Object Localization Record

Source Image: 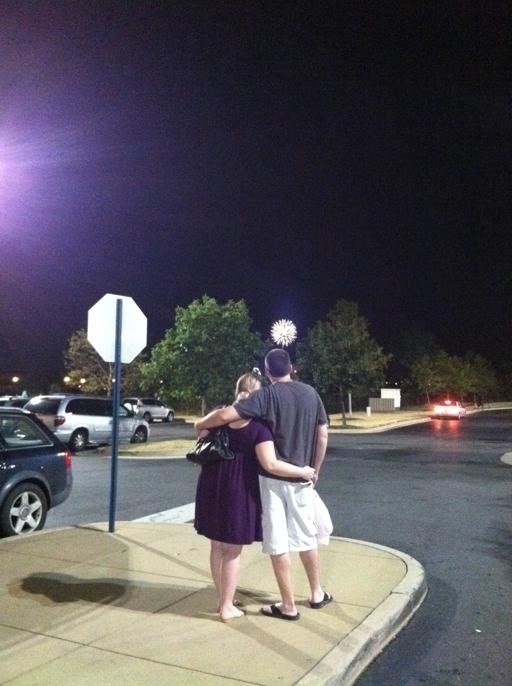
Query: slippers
[259,602,298,621]
[309,591,333,608]
[218,598,247,621]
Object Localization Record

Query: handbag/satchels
[186,427,236,465]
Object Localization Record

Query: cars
[431,400,467,420]
[0,392,179,450]
[0,404,76,537]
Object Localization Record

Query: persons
[193,349,333,621]
[193,374,316,624]
